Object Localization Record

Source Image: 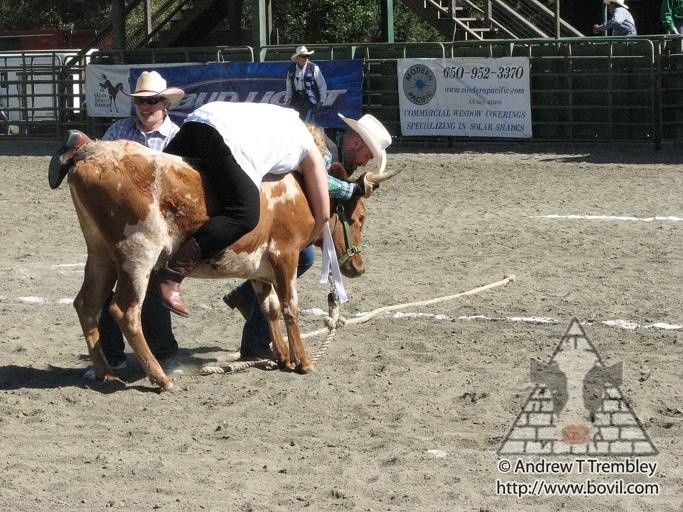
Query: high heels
[222,280,257,321]
[47,129,93,190]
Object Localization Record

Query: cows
[66,138,408,396]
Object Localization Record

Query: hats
[603,0,629,10]
[338,112,393,174]
[290,45,315,63]
[121,70,185,104]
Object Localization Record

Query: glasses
[134,97,163,105]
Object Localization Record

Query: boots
[150,236,204,318]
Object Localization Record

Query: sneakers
[83,359,128,380]
[159,358,185,377]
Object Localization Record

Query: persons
[83,70,185,382]
[659,0,683,72]
[149,99,332,318]
[222,111,393,361]
[284,45,328,123]
[592,0,638,44]
